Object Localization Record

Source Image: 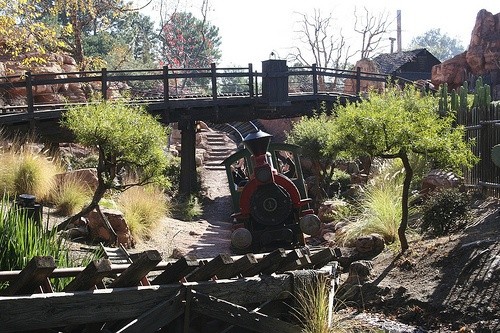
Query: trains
[222,127,321,254]
